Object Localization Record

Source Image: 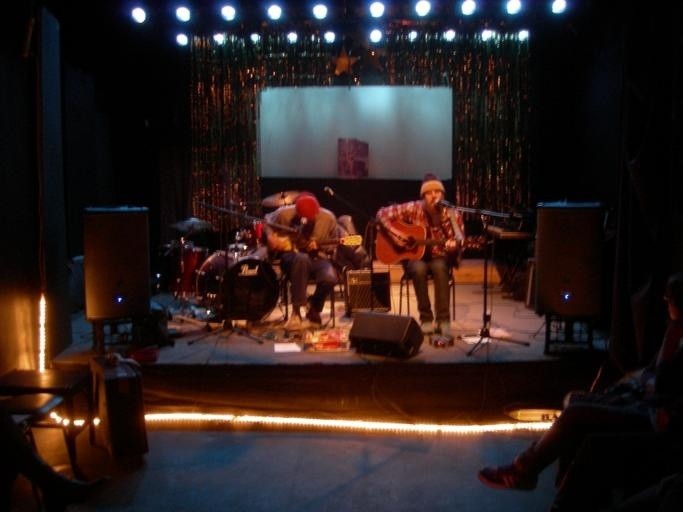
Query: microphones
[434,198,457,210]
[294,216,310,233]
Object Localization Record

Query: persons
[0,407,111,512]
[264,196,338,331]
[377,173,465,334]
[478,281,683,492]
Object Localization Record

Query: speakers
[130,310,168,348]
[349,312,424,358]
[346,270,391,318]
[533,202,607,321]
[83,207,152,320]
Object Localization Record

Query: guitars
[267,230,363,260]
[376,222,481,265]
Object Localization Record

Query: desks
[0,368,95,482]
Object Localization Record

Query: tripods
[188,203,296,345]
[457,235,531,357]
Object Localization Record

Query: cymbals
[262,191,302,207]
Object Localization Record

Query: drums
[195,251,278,320]
[174,248,211,301]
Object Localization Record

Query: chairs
[397,267,455,324]
[281,274,336,329]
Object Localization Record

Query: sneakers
[477,454,538,491]
[284,308,323,329]
[421,318,449,339]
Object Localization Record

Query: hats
[418,172,446,196]
[296,191,320,218]
